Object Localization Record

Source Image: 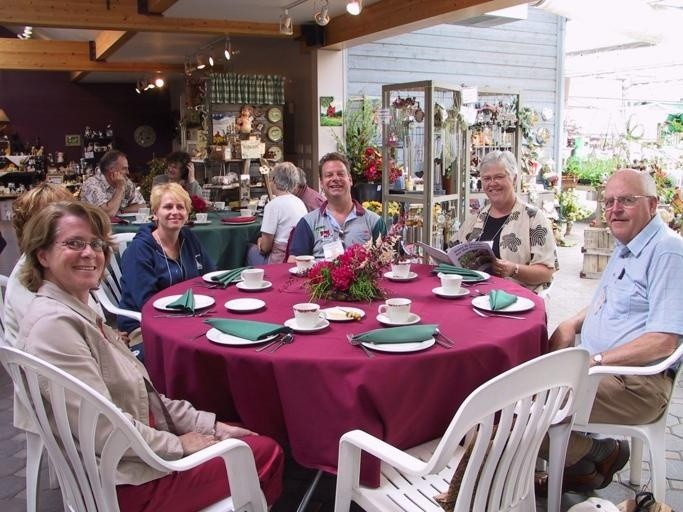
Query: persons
[517,169,683,497]
[287,153,387,264]
[151,155,205,216]
[248,160,327,267]
[116,181,214,353]
[83,155,144,222]
[4,186,132,372]
[448,151,554,295]
[12,200,284,512]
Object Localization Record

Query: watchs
[593,352,605,366]
[510,263,520,278]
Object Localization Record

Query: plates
[131,220,151,225]
[288,267,306,276]
[236,280,273,291]
[432,286,471,298]
[134,125,156,148]
[267,108,283,162]
[319,306,366,322]
[202,270,242,282]
[437,270,491,283]
[192,220,212,225]
[225,298,266,312]
[152,294,216,312]
[359,335,436,352]
[383,271,419,281]
[375,313,421,326]
[284,317,330,333]
[206,327,279,346]
[471,295,535,312]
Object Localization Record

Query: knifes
[462,283,490,287]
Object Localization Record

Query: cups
[438,273,463,293]
[391,259,411,278]
[241,268,265,287]
[378,297,412,322]
[295,255,316,272]
[136,202,257,222]
[292,303,326,328]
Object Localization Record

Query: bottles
[26,124,113,188]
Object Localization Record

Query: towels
[488,289,517,312]
[433,263,483,282]
[352,323,439,344]
[221,215,255,223]
[203,316,297,342]
[112,216,129,226]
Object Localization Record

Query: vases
[358,183,382,203]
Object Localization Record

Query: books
[414,239,496,282]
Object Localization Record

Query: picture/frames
[65,134,80,147]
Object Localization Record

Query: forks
[346,332,376,359]
[473,308,527,321]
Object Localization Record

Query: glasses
[602,195,654,209]
[53,239,109,253]
[478,174,508,183]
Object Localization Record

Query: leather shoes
[535,465,604,496]
[597,439,631,490]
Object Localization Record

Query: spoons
[270,332,294,352]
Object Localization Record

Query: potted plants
[561,160,580,192]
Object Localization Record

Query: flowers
[329,96,402,183]
[187,195,214,219]
[307,202,446,301]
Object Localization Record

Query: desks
[142,257,547,512]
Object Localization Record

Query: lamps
[136,73,165,92]
[184,36,239,74]
[280,0,364,35]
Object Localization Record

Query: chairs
[571,339,683,503]
[335,346,589,512]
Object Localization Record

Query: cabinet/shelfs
[382,81,534,251]
